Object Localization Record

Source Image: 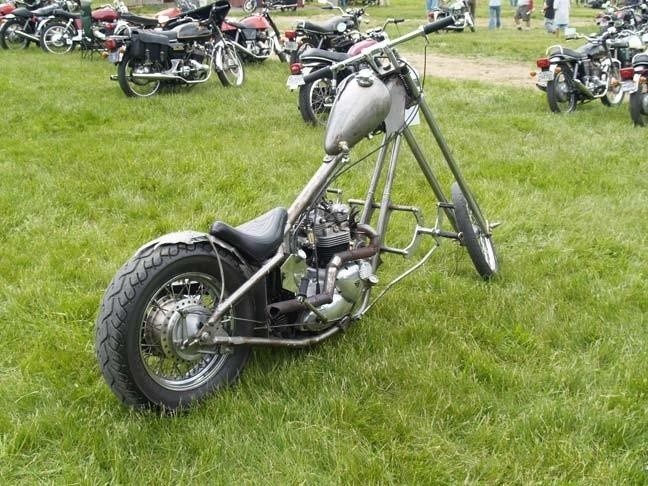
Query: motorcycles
[530,0,648,124]
[427,0,475,35]
[0,0,404,128]
[94,15,500,417]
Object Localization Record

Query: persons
[515,0,533,31]
[487,0,502,31]
[553,0,570,38]
[539,0,558,34]
